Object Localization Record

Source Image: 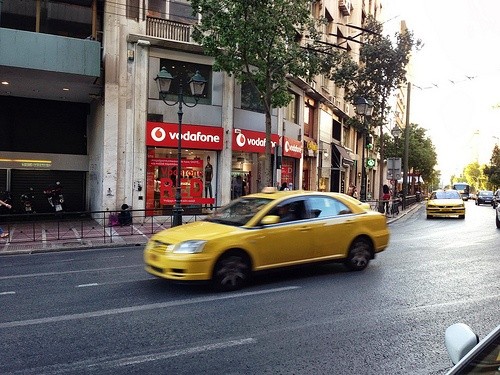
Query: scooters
[18,188,36,221]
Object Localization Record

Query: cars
[426,190,465,220]
[142,187,391,290]
[493,189,500,209]
[442,323,500,374]
[496,208,500,227]
[475,191,493,205]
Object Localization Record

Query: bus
[454,183,469,201]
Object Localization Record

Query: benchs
[377,198,403,214]
[405,195,416,210]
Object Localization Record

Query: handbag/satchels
[108,212,120,226]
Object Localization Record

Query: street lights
[153,65,207,224]
[353,96,376,199]
[391,123,400,201]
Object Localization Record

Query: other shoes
[0,232,9,238]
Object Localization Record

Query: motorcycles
[42,181,65,222]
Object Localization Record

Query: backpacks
[383,194,390,200]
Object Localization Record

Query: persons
[280,182,289,191]
[233,176,244,199]
[201,155,213,211]
[0,198,13,238]
